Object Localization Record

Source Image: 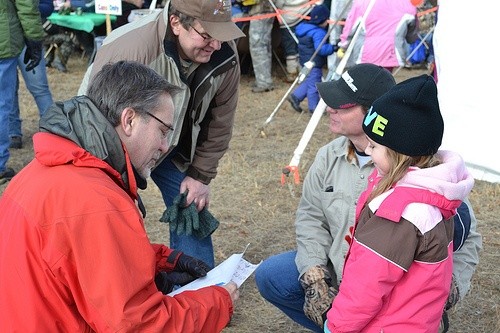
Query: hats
[308,5,329,25]
[171,0,246,42]
[362,74,444,156]
[315,63,396,110]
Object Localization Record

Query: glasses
[145,110,174,141]
[188,23,225,44]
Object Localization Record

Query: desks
[45,11,117,65]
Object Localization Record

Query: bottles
[76,7,82,16]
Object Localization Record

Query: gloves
[333,45,339,51]
[299,264,338,329]
[175,254,214,279]
[23,38,43,71]
[159,190,221,241]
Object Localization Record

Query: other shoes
[50,61,66,72]
[0,167,16,185]
[286,72,298,82]
[252,87,275,92]
[288,94,303,113]
[9,135,22,147]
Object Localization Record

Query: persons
[231,0,438,115]
[0,60,240,333]
[78,0,246,293]
[0,0,153,186]
[323,75,475,333]
[255,63,484,333]
